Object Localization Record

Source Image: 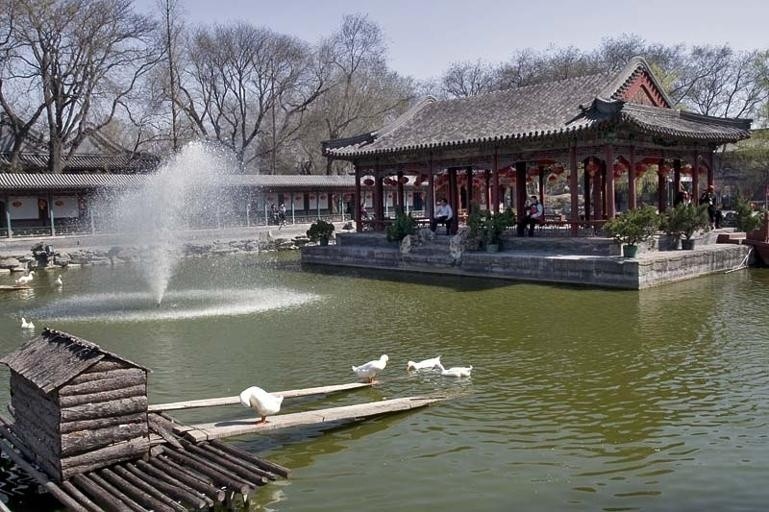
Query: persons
[516,196,544,237]
[270,204,280,225]
[278,203,288,225]
[673,183,722,229]
[430,198,453,236]
[361,202,376,231]
[749,201,761,214]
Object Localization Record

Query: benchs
[364,215,607,238]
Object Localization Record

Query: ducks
[406,355,442,371]
[21,317,35,329]
[15,271,36,287]
[239,385,283,425]
[55,274,63,286]
[352,354,389,383]
[432,363,473,377]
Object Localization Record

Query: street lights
[667,178,673,207]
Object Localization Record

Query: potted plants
[600,204,660,257]
[659,200,710,249]
